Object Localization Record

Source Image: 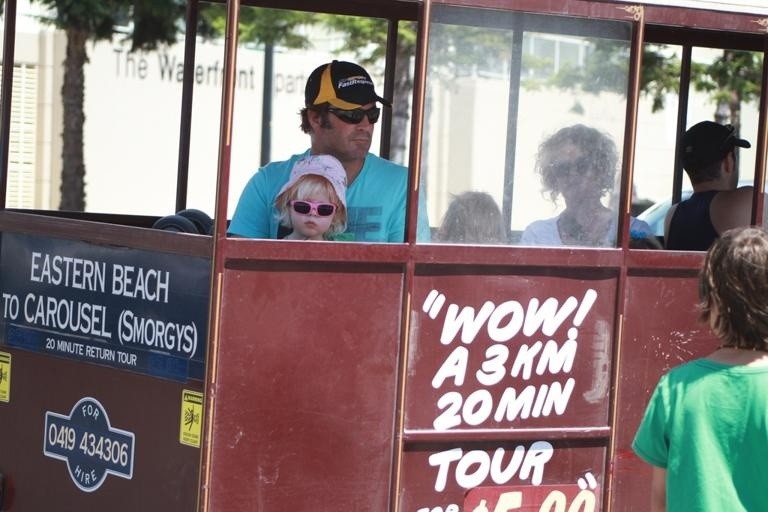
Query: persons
[518,125,663,248]
[663,121,767,251]
[226,59,431,245]
[435,190,510,244]
[627,226,768,511]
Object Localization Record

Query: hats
[677,121,751,169]
[304,60,392,112]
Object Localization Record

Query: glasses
[290,199,337,220]
[547,156,594,176]
[721,123,736,144]
[329,108,381,124]
